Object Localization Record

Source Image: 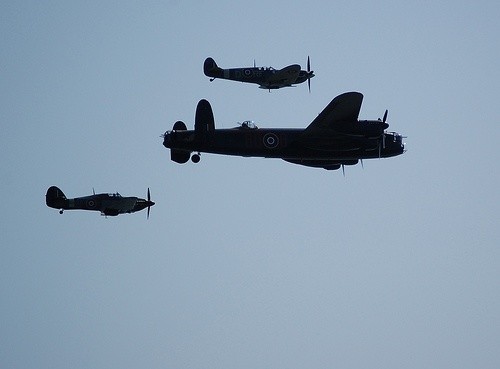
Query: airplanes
[203,55,315,92]
[160,91,407,175]
[46,186,155,219]
